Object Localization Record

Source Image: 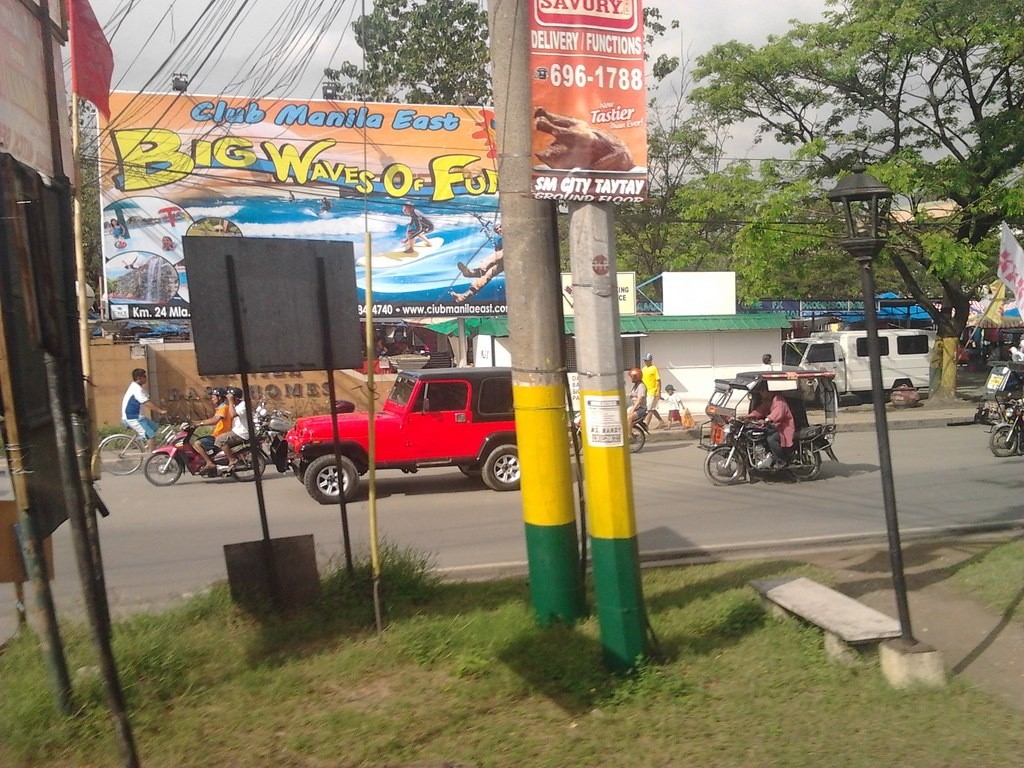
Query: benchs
[747,575,902,659]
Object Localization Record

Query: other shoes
[451,291,470,304]
[227,459,239,472]
[773,456,789,469]
[458,262,474,277]
[655,423,671,430]
[199,464,216,474]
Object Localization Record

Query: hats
[643,353,652,361]
[665,385,674,390]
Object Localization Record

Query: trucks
[782,330,935,395]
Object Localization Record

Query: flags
[997,220,1024,324]
[67,0,114,123]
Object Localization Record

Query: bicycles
[98,413,180,476]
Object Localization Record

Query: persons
[761,354,774,371]
[450,221,505,305]
[957,334,1024,373]
[109,219,127,239]
[737,382,795,469]
[363,329,421,358]
[641,353,665,431]
[189,385,231,473]
[660,384,689,430]
[120,368,169,465]
[625,367,647,440]
[402,202,435,253]
[223,387,249,473]
[161,237,177,252]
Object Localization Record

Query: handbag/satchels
[681,409,696,428]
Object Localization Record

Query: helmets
[209,387,242,400]
[628,368,642,382]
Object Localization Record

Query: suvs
[285,367,521,505]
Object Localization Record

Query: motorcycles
[143,422,269,487]
[704,415,838,486]
[567,412,650,456]
[254,400,354,446]
[990,393,1024,456]
[981,361,1024,424]
[700,374,839,451]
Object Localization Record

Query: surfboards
[356,237,444,268]
[309,207,320,217]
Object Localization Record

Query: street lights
[823,161,914,642]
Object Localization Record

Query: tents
[930,298,1024,337]
